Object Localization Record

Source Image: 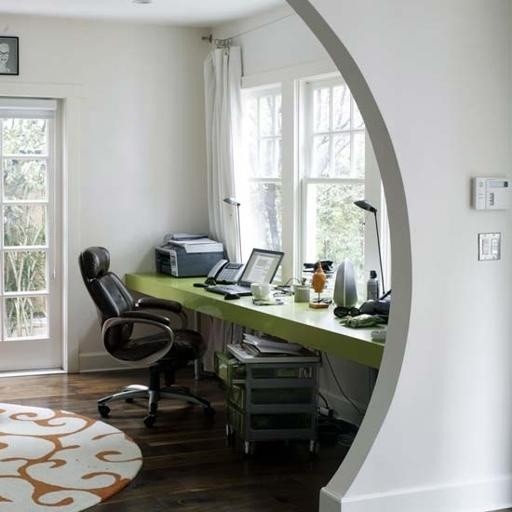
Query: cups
[251,282,270,300]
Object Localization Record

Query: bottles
[368,270,379,301]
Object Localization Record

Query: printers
[155,242,224,278]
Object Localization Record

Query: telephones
[207,259,244,284]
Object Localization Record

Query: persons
[0,43,10,73]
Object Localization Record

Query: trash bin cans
[315,419,359,476]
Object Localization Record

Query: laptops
[207,248,284,296]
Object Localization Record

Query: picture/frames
[0,35,23,77]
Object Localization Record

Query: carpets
[0,402,144,512]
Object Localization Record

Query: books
[225,332,324,365]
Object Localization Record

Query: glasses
[334,307,360,318]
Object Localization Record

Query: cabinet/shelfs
[214,338,322,461]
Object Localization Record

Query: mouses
[225,293,240,300]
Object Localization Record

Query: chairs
[78,247,219,426]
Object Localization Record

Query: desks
[124,247,389,381]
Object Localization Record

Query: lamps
[224,196,245,278]
[350,198,387,314]
